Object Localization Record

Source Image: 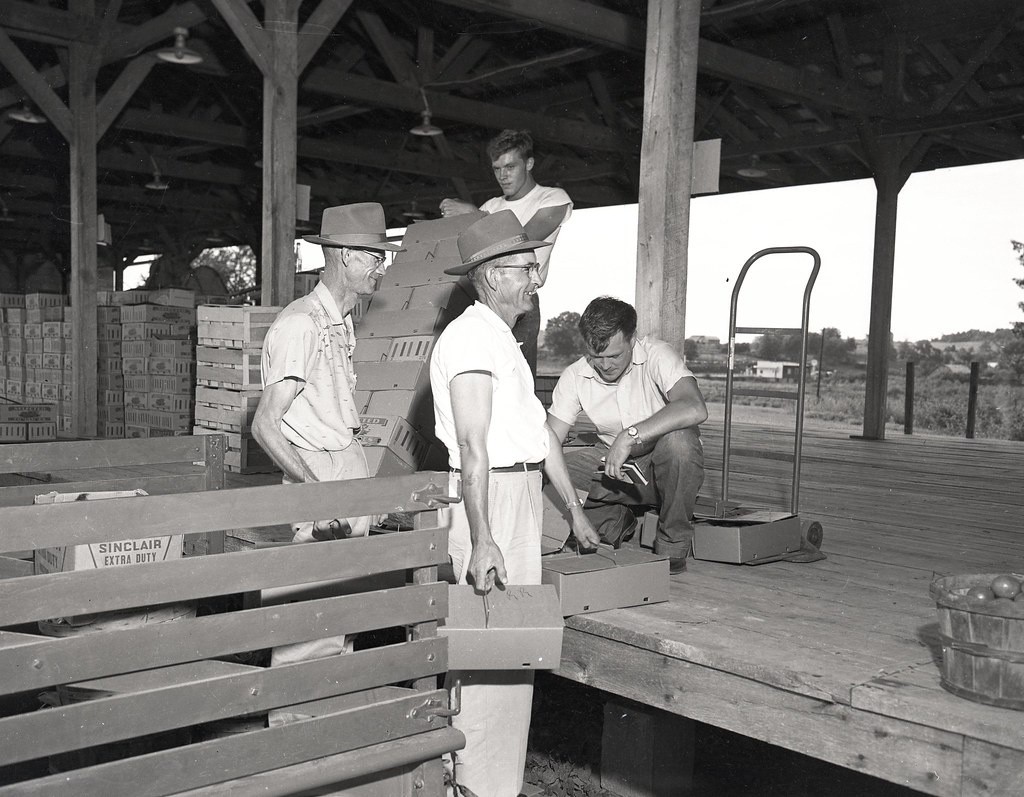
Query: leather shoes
[600,505,638,551]
[668,557,686,574]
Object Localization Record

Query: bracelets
[564,497,585,512]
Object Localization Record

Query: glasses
[360,248,386,267]
[494,263,541,278]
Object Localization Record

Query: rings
[440,209,445,216]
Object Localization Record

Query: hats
[302,203,406,252]
[444,209,553,276]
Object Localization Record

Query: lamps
[8,99,48,125]
[402,201,426,218]
[137,238,155,250]
[156,26,204,65]
[736,156,769,178]
[408,88,445,136]
[205,228,225,243]
[145,154,172,190]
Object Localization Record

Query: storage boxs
[0,209,489,480]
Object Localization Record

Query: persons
[437,125,573,398]
[247,200,392,735]
[427,203,603,797]
[541,289,709,576]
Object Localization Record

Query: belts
[449,458,545,474]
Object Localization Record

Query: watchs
[627,425,644,447]
